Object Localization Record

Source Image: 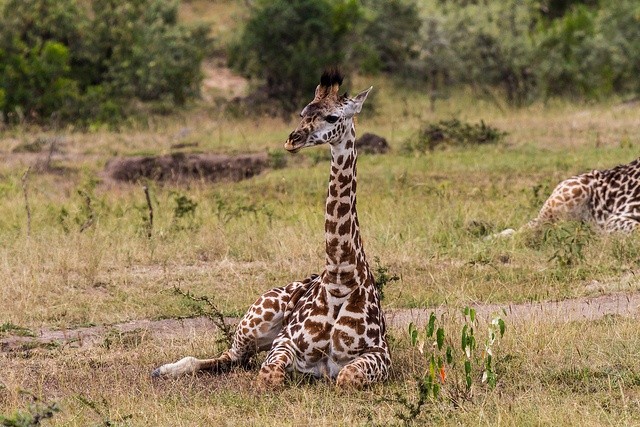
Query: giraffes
[150,65,394,397]
[484,156,640,249]
